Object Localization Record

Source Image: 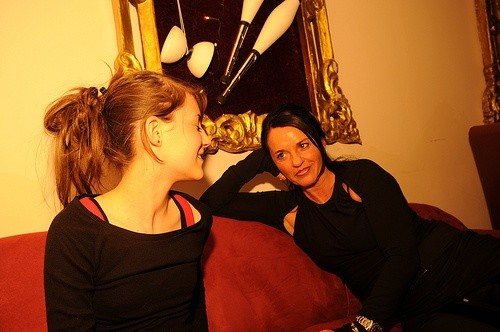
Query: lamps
[160,0,217,78]
[216,0,300,106]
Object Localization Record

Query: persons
[43,50,212,332]
[197,101,500,331]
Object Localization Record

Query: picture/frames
[112,0,362,154]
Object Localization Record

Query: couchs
[1,203,500,332]
